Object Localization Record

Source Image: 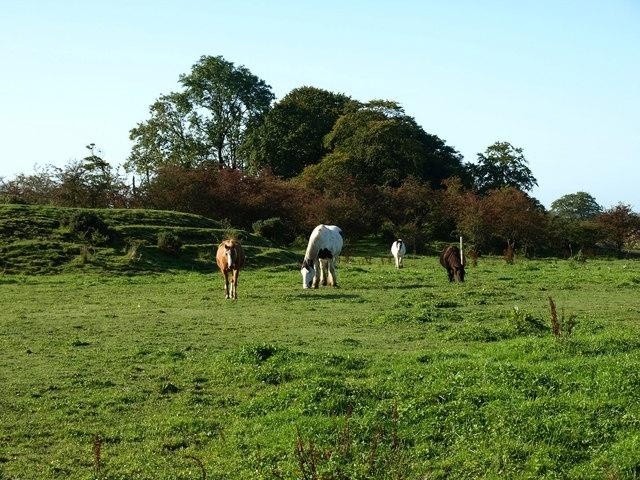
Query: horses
[216,239,243,301]
[439,245,465,282]
[298,224,344,289]
[391,239,406,269]
